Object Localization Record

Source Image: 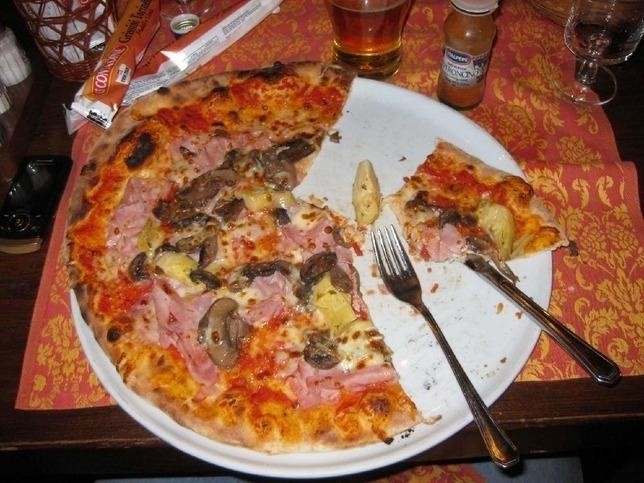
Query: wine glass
[553,0,642,104]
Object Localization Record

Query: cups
[324,2,412,78]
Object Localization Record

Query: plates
[70,77,553,481]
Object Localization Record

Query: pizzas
[66,60,425,455]
[383,137,571,283]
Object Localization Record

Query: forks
[370,224,527,478]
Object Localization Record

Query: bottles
[437,1,500,110]
